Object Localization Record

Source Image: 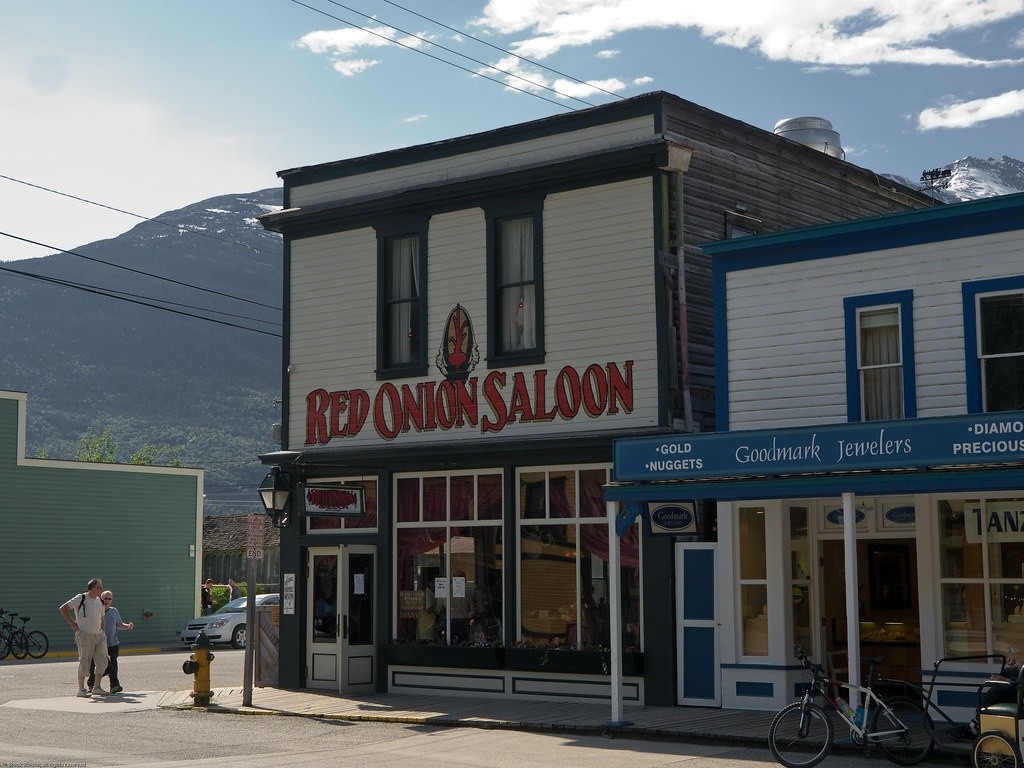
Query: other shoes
[110,686,122,694]
[87,679,92,692]
[92,686,110,695]
[944,724,979,743]
[77,689,92,697]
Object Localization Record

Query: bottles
[855,704,864,725]
[836,697,856,717]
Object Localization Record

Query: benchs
[976,664,1024,756]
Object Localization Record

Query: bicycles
[768,642,936,768]
[1,610,49,659]
[0,607,29,660]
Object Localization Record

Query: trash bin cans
[254,605,280,688]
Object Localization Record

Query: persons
[944,658,1024,743]
[582,599,601,640]
[86,590,135,694]
[416,570,490,645]
[57,577,110,697]
[598,597,605,631]
[858,584,865,620]
[201,579,213,615]
[228,577,240,602]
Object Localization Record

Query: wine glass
[554,637,559,649]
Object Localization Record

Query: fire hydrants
[181,628,215,708]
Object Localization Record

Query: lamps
[256,466,292,528]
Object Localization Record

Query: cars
[180,593,280,649]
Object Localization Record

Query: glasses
[95,587,103,589]
[104,598,112,600]
